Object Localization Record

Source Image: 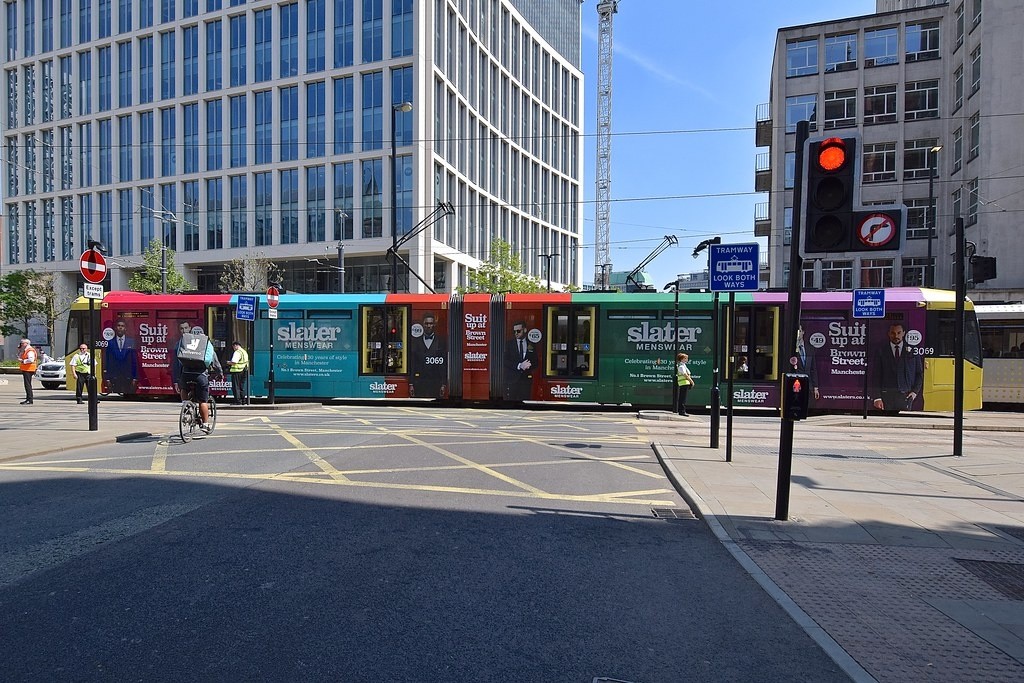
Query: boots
[77,397,84,404]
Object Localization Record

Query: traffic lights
[804,137,856,252]
[783,373,809,419]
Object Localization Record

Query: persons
[178,326,225,433]
[677,353,694,417]
[872,323,923,410]
[504,322,539,401]
[408,315,447,399]
[17,338,37,404]
[171,320,192,393]
[39,350,51,363]
[105,321,137,394]
[226,342,248,405]
[793,324,820,399]
[69,344,100,404]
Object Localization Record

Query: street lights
[537,253,561,293]
[392,100,414,293]
[332,207,349,293]
[926,144,943,289]
[594,264,613,290]
[152,214,180,293]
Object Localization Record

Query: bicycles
[179,382,217,443]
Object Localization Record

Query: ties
[895,346,900,368]
[119,337,122,352]
[798,346,806,367]
[520,340,524,362]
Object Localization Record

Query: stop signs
[79,249,107,283]
[266,286,280,308]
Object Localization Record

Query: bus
[101,247,985,413]
[973,301,1024,411]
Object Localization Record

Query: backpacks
[177,333,213,368]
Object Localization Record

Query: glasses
[512,330,524,333]
[80,348,86,350]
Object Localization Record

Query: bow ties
[425,336,433,340]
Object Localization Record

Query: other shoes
[20,400,33,404]
[242,401,247,405]
[201,425,210,433]
[230,400,242,405]
[680,412,689,416]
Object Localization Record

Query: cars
[34,355,66,388]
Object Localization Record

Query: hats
[190,326,204,334]
